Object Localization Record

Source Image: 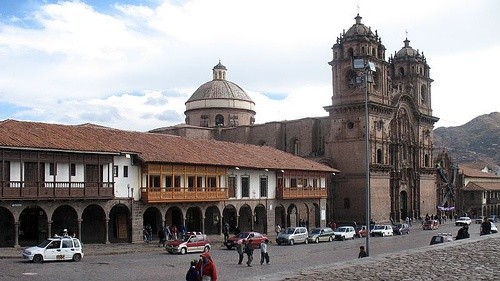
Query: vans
[276,227,309,245]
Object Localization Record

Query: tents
[438,201,455,219]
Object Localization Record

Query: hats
[200,252,209,259]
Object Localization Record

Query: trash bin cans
[430,236,443,245]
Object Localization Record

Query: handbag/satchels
[201,275,212,281]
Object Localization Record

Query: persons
[158,225,187,248]
[261,239,270,265]
[224,221,230,241]
[299,219,305,227]
[246,239,254,267]
[326,221,336,231]
[353,222,357,227]
[445,211,457,221]
[482,217,491,235]
[234,227,240,235]
[186,252,217,281]
[425,212,438,222]
[218,222,221,235]
[358,246,367,258]
[455,223,469,240]
[275,224,281,236]
[143,225,152,241]
[406,216,412,226]
[237,240,246,265]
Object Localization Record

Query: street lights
[351,55,385,257]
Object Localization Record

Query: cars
[22,234,84,263]
[422,220,440,230]
[356,225,393,238]
[487,215,497,223]
[308,228,336,244]
[455,217,472,226]
[430,233,454,245]
[475,216,486,224]
[163,232,211,255]
[334,226,356,241]
[394,223,410,235]
[480,222,498,235]
[224,231,270,251]
